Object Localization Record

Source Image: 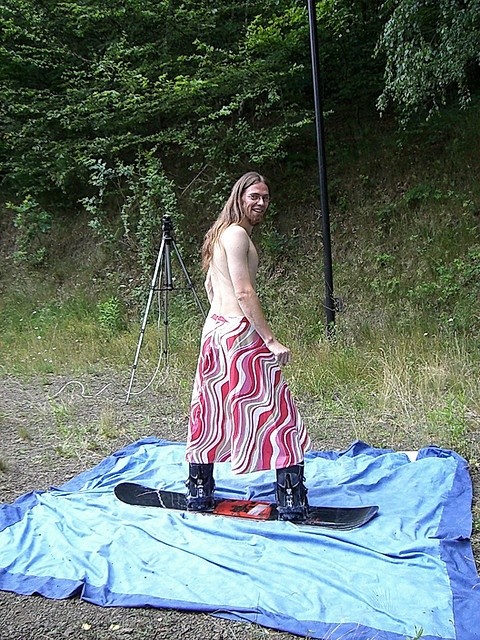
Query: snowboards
[114,482,379,531]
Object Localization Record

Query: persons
[183,171,311,523]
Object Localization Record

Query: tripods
[124,214,207,404]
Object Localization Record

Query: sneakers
[276,494,308,522]
[184,481,216,510]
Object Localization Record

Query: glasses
[241,193,272,202]
